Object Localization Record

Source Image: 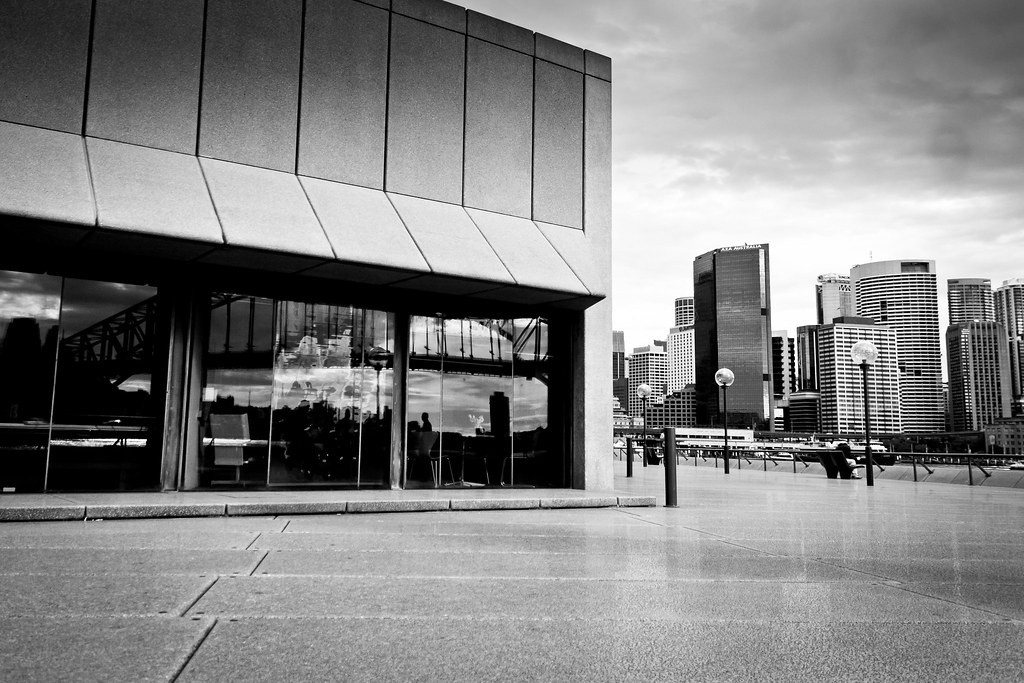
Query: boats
[1009,462,1024,470]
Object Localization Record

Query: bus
[664,427,753,448]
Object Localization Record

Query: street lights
[637,385,651,467]
[715,367,734,474]
[850,340,879,486]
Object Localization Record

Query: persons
[419,412,433,432]
[335,408,356,439]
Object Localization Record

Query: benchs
[817,451,865,479]
[646,448,662,465]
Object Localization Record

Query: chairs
[500,426,549,486]
[409,432,454,489]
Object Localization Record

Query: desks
[457,434,495,487]
